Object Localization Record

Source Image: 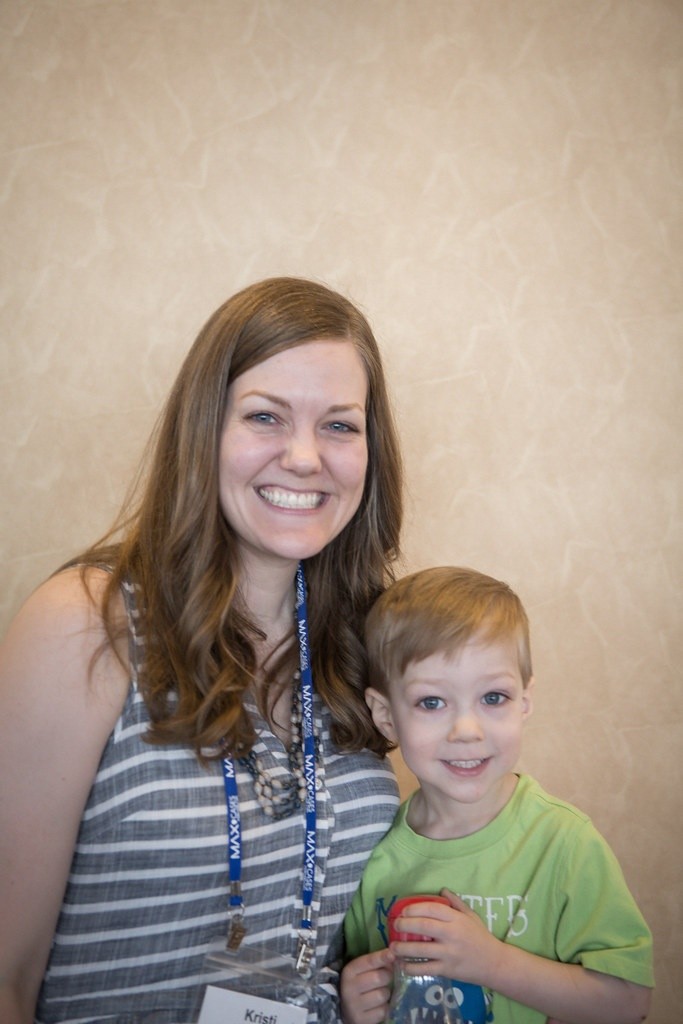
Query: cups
[388,895,452,962]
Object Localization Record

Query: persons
[1,274,404,1024]
[337,565,656,1024]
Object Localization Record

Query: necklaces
[235,664,305,822]
